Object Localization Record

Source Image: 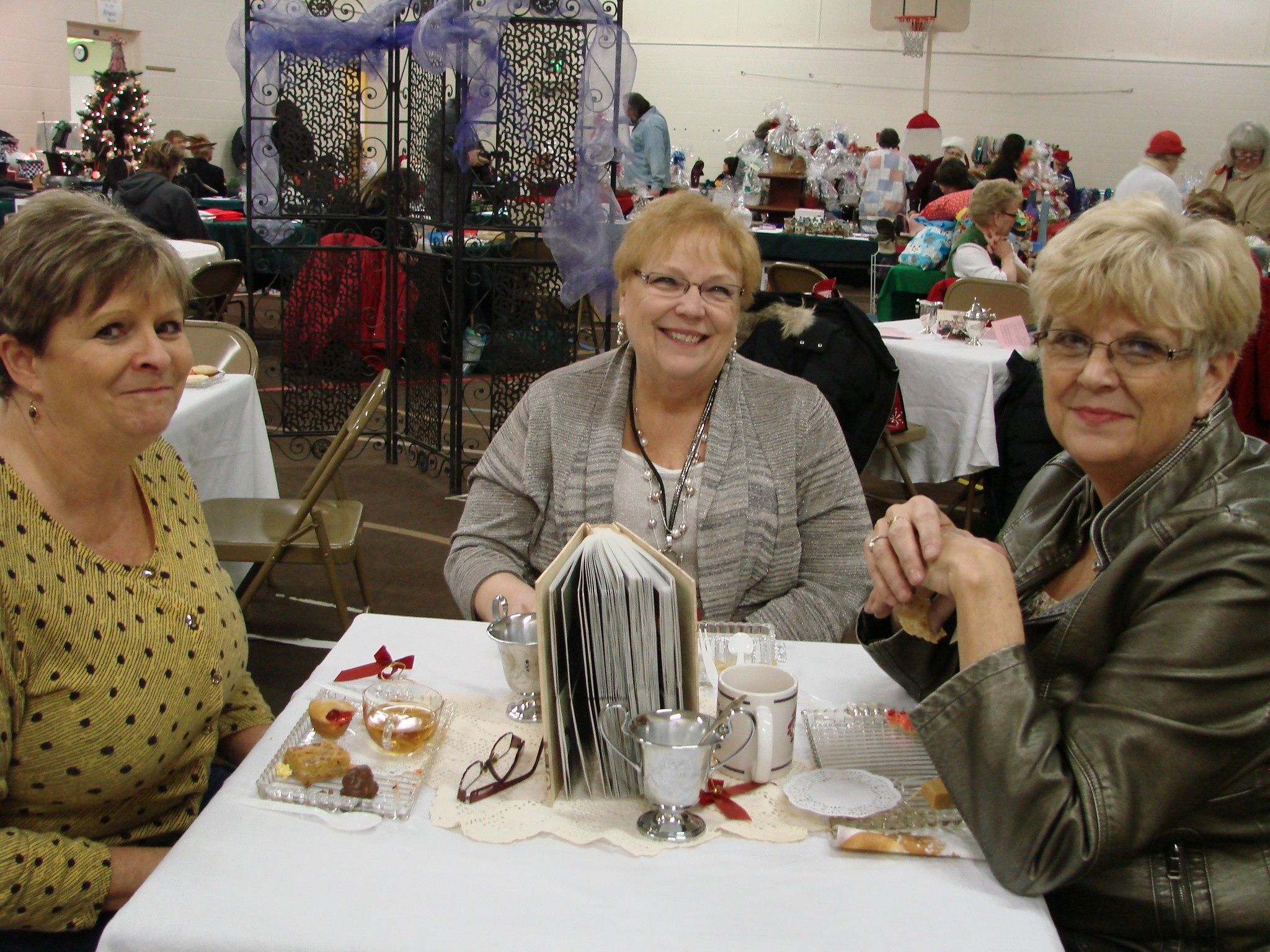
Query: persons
[442,193,871,644]
[0,188,276,952]
[857,196,1269,952]
[112,94,1269,307]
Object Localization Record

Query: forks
[697,628,718,690]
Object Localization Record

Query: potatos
[308,699,357,739]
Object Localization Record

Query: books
[536,520,699,808]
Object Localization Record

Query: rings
[868,534,886,552]
[886,516,909,527]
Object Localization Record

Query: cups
[362,680,444,757]
[713,664,799,783]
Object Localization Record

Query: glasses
[633,268,746,308]
[456,731,545,805]
[1231,150,1261,161]
[1000,210,1020,221]
[1030,328,1194,375]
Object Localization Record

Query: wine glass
[916,298,944,335]
[598,696,757,842]
[954,297,996,346]
[487,593,542,724]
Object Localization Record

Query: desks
[752,222,914,274]
[855,315,1041,485]
[94,612,1065,952]
[157,373,283,594]
[163,193,321,320]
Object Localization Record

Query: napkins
[828,822,987,860]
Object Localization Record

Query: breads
[341,764,378,799]
[284,739,351,786]
[922,778,952,810]
[895,592,948,643]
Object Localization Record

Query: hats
[184,132,218,150]
[1051,150,1072,163]
[1146,131,1187,155]
[941,136,966,151]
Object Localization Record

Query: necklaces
[633,378,710,566]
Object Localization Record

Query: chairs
[201,368,390,633]
[767,262,1037,531]
[181,236,259,379]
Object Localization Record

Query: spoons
[234,798,383,832]
[728,631,754,664]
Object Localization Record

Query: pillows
[898,188,972,272]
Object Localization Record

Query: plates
[783,768,902,817]
[801,703,963,832]
[697,619,787,686]
[184,370,225,388]
